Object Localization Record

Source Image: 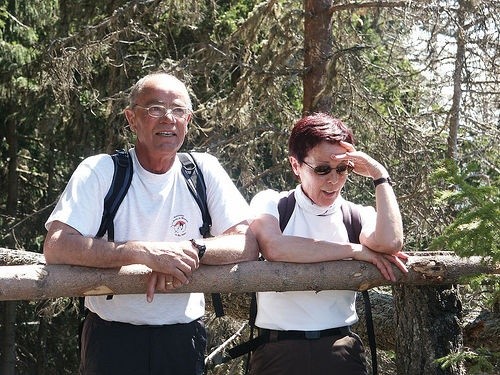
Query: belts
[211,325,352,366]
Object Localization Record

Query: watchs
[190,238,206,260]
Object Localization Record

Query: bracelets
[373,176,393,188]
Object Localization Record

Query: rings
[165,281,172,285]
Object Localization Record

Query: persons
[249,114,409,375]
[43,73,261,375]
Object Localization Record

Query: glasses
[294,156,355,175]
[131,103,191,119]
